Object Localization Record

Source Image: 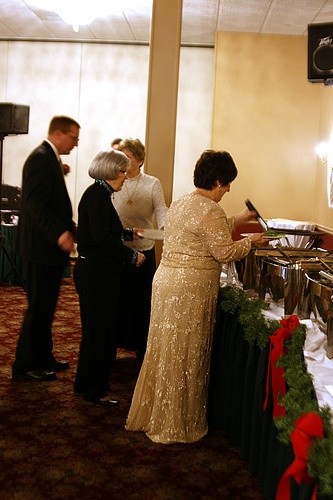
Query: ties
[59,160,64,176]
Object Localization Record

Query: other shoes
[95,397,118,407]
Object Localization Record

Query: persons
[13,116,81,380]
[126,149,270,452]
[74,137,170,405]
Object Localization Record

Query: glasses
[119,170,127,176]
[63,132,79,142]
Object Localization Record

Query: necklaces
[125,171,142,204]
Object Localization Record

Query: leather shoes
[12,369,56,381]
[48,359,70,371]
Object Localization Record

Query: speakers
[307,22,333,85]
[0,102,29,135]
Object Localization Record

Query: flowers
[63,163,70,175]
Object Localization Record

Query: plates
[137,229,164,240]
[240,233,285,239]
